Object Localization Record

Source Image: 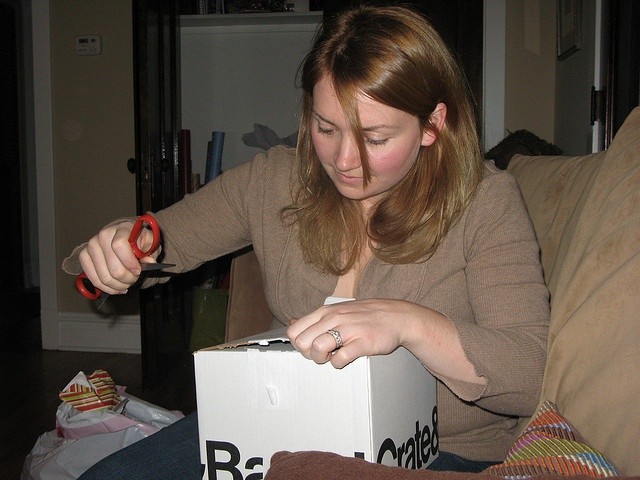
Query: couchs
[226,106,640,479]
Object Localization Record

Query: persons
[62,1,551,479]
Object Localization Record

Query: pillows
[482,400,622,477]
[266,450,500,480]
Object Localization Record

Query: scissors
[76,215,177,311]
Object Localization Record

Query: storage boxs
[194,328,440,480]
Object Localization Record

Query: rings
[326,329,343,349]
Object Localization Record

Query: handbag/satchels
[19,388,185,479]
